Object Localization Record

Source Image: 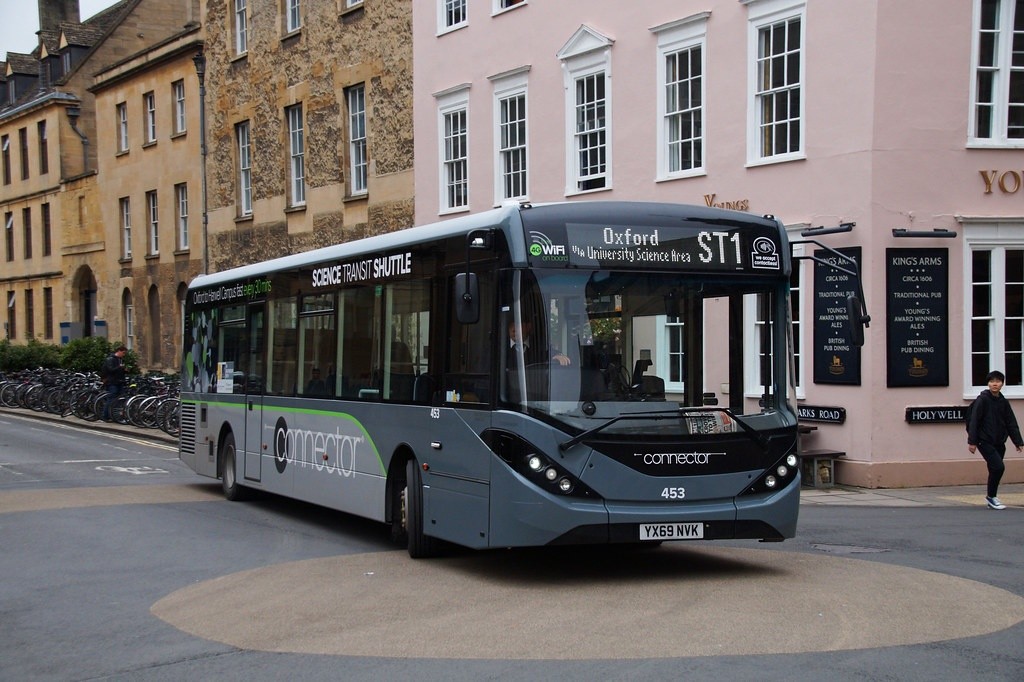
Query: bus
[176,198,872,561]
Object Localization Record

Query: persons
[99,346,129,424]
[378,323,416,400]
[488,305,571,370]
[327,336,368,385]
[965,370,1024,510]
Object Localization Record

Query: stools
[796,447,846,488]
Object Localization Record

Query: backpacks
[965,392,992,436]
[101,355,119,379]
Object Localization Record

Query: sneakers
[985,496,1006,509]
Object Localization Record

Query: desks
[797,424,818,474]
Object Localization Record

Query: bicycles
[0,366,179,437]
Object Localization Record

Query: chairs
[214,325,415,405]
